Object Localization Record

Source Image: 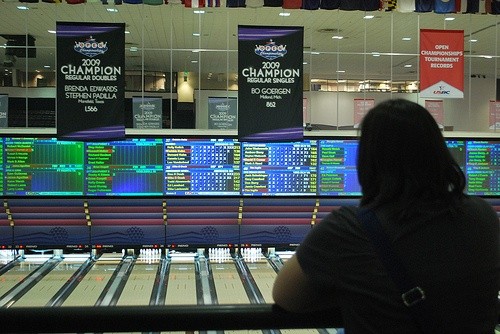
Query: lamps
[177,65,194,102]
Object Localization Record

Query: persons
[273,98,500,334]
